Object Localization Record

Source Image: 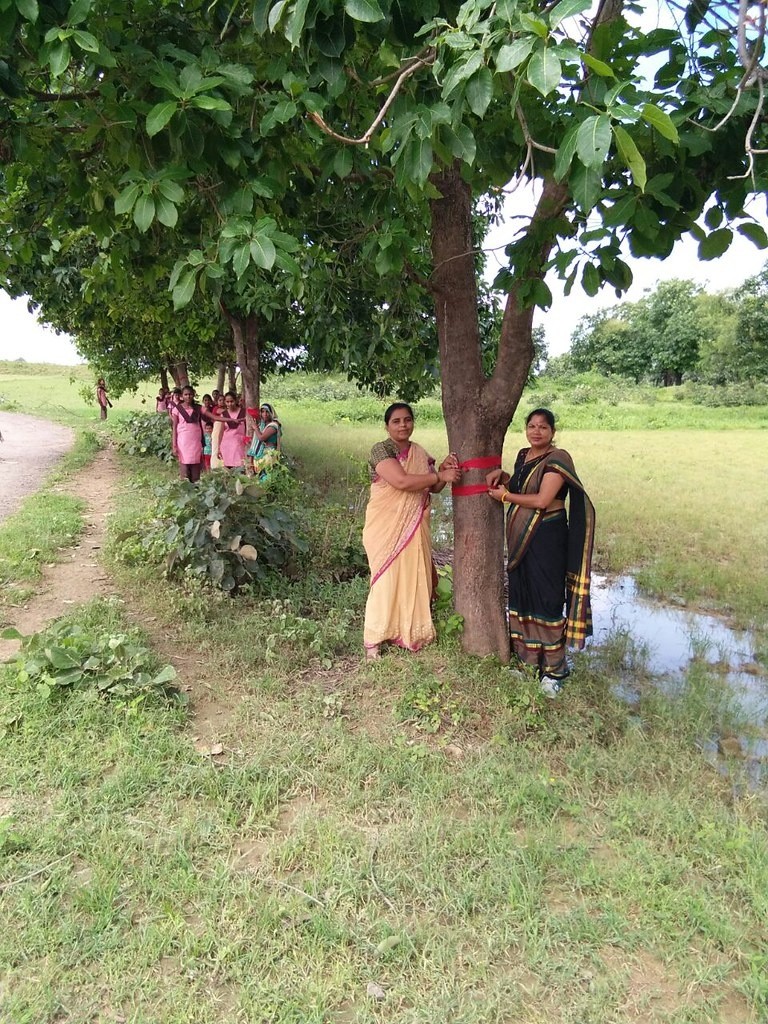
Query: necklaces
[518,445,552,495]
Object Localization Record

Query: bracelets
[434,472,439,483]
[502,492,510,504]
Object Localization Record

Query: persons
[98,378,113,419]
[485,409,596,700]
[248,404,283,485]
[171,386,245,483]
[217,391,249,474]
[200,390,226,473]
[362,403,462,661]
[156,387,184,420]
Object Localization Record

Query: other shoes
[541,676,563,699]
[367,645,381,664]
[508,669,524,680]
